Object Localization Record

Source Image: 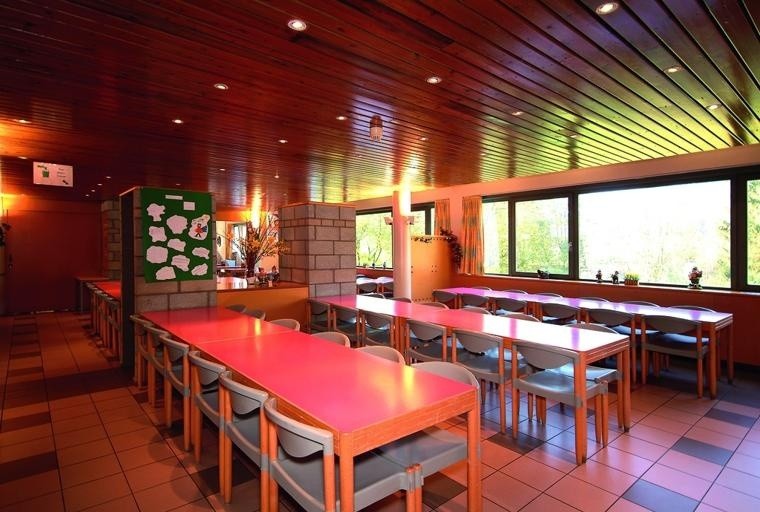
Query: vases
[688,279,702,289]
[246,262,256,284]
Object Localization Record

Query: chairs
[356,274,394,296]
[86,279,123,364]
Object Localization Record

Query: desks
[73,273,111,314]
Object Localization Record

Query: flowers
[216,210,292,266]
[688,266,703,280]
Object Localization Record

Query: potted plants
[624,272,641,285]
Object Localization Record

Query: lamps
[369,114,383,141]
